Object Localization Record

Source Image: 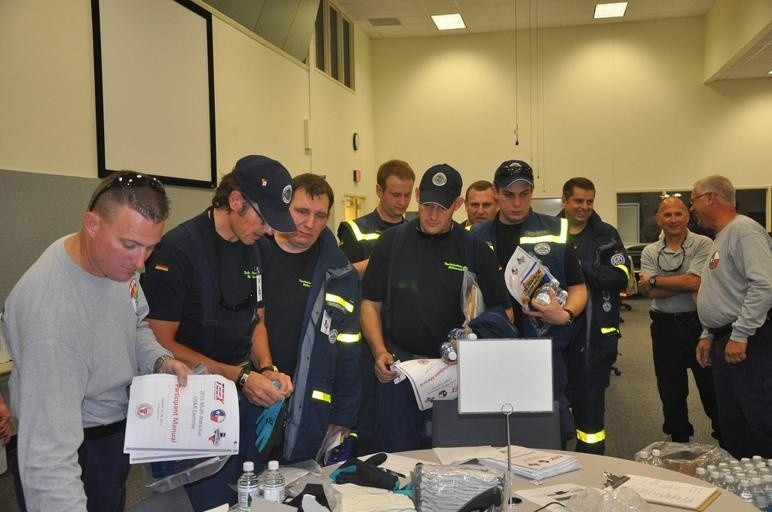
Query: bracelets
[234,363,252,392]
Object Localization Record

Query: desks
[227,446,762,512]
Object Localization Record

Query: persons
[638,197,719,444]
[5,168,193,511]
[255,174,364,464]
[140,156,298,512]
[690,176,772,456]
[335,159,632,457]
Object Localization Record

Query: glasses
[660,192,683,202]
[89,172,167,212]
[689,191,717,206]
[237,191,267,228]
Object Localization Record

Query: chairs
[617,254,633,311]
[124,486,194,512]
[431,399,562,451]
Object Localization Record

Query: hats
[495,160,534,193]
[419,164,462,211]
[232,154,299,237]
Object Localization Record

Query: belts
[650,310,695,325]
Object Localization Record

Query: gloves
[330,456,400,490]
[255,381,287,458]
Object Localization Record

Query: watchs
[649,274,659,287]
[258,365,278,375]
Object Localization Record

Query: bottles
[648,448,665,467]
[534,278,569,308]
[438,342,458,362]
[327,433,358,466]
[637,450,650,465]
[447,327,477,342]
[694,455,771,512]
[238,462,260,512]
[263,461,287,505]
